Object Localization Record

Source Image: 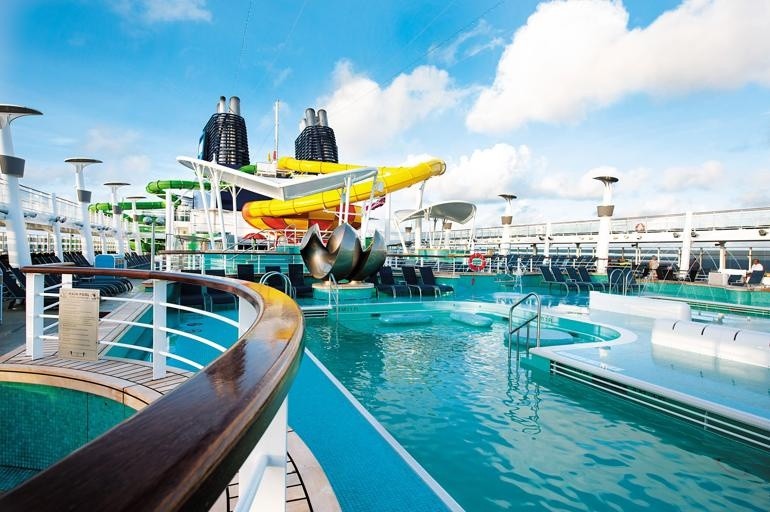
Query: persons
[744,258,764,283]
[648,254,660,278]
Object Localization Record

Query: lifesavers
[635,223,645,233]
[468,253,486,270]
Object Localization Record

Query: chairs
[0,251,151,311]
[748,269,765,284]
[376,254,644,300]
[176,265,312,321]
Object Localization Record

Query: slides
[242,156,446,244]
[88,163,256,254]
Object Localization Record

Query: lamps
[0,207,117,234]
